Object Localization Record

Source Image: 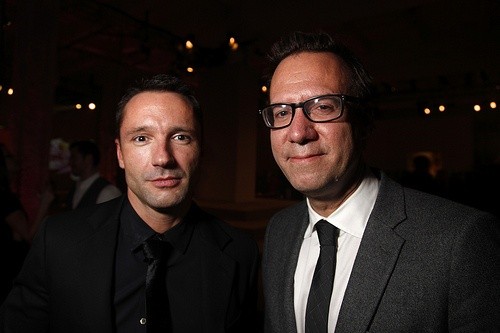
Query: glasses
[259,94,363,129]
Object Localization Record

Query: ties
[143,233,173,333]
[305,220,340,333]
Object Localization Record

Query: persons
[0,74,261,333]
[63,136,121,209]
[0,142,58,304]
[379,98,500,216]
[256,31,500,333]
